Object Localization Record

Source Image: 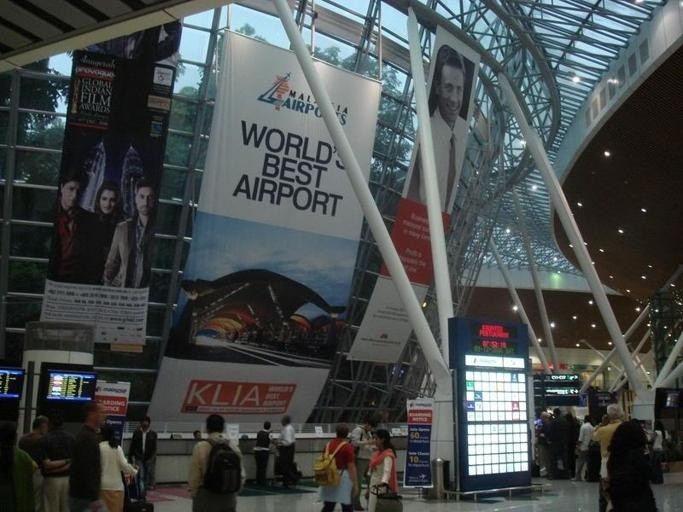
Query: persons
[419,45,468,214]
[363,429,398,512]
[271,413,297,489]
[253,419,279,489]
[193,430,200,438]
[46,169,100,283]
[0,402,158,511]
[536,404,674,511]
[101,179,157,288]
[346,416,378,511]
[185,413,247,512]
[314,422,360,511]
[91,178,128,259]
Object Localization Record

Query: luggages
[375,492,403,512]
[124,496,154,512]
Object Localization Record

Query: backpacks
[311,439,351,487]
[198,438,242,496]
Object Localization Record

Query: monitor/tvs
[42,370,95,403]
[0,368,26,401]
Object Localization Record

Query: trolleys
[120,464,155,512]
[254,449,302,488]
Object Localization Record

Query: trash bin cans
[432,458,450,499]
